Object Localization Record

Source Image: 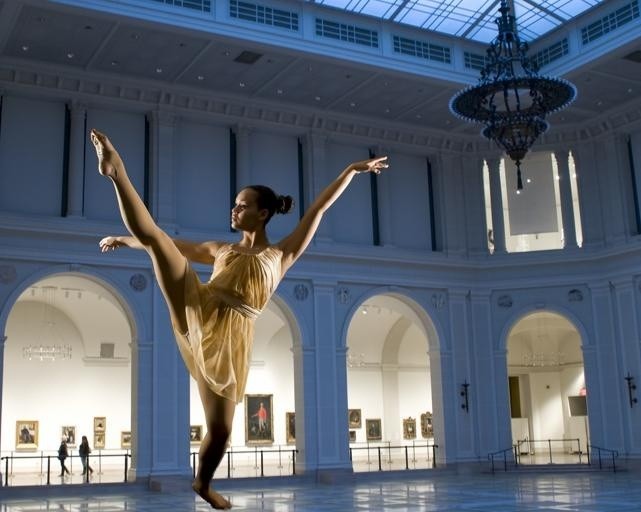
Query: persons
[21,426,32,443]
[89,129,389,509]
[79,436,93,475]
[369,423,378,436]
[251,403,266,432]
[58,442,71,476]
[64,427,73,442]
[190,427,200,441]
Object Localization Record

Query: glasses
[448,1,579,189]
[519,351,568,366]
[346,352,370,368]
[23,343,73,363]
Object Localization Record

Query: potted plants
[121,432,131,449]
[62,426,77,448]
[15,421,38,452]
[348,409,362,427]
[402,411,434,440]
[244,395,274,447]
[366,419,382,440]
[94,417,106,448]
[190,425,203,444]
[286,412,295,446]
